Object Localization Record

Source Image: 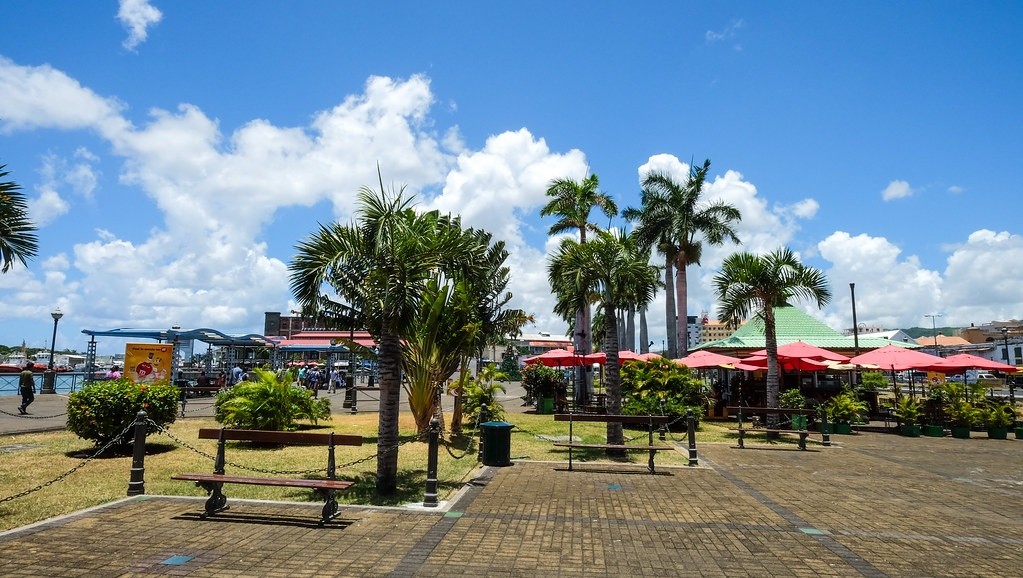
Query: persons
[17,362,36,414]
[105,365,121,381]
[195,366,347,399]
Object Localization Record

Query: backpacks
[237,373,246,382]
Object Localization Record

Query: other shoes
[196,393,199,396]
[18,407,24,413]
[201,393,204,396]
[21,412,28,414]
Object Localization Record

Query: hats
[314,366,319,369]
[305,366,308,368]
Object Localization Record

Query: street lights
[39,307,65,394]
[1002,328,1016,408]
[924,314,943,377]
[902,336,913,399]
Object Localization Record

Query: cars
[977,374,996,378]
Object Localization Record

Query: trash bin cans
[367,375,374,387]
[480,421,516,467]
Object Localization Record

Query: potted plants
[778,371,1023,440]
[536,366,559,415]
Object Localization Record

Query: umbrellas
[287,361,318,368]
[522,338,1018,403]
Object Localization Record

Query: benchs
[553,412,674,474]
[172,427,362,528]
[726,406,821,451]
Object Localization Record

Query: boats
[0,339,73,373]
